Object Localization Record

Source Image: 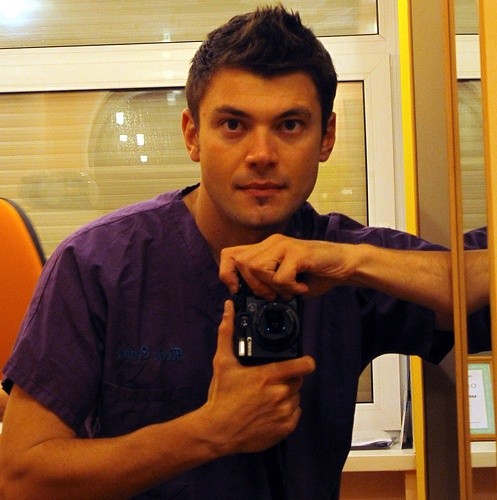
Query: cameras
[233,272,303,366]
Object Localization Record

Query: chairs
[0,197,44,380]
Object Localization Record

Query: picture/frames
[467,355,496,442]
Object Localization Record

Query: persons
[0,9,454,499]
[463,226,491,355]
